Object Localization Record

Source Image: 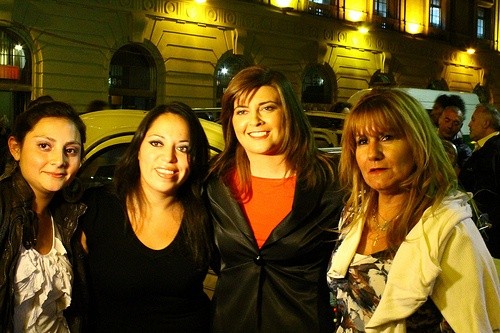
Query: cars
[52,108,224,228]
[302,110,348,148]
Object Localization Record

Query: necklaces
[372,211,399,246]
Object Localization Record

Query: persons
[74,101,209,333]
[326,88,500,333]
[426,93,500,259]
[0,94,88,333]
[196,66,349,333]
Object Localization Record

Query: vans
[346,86,481,140]
